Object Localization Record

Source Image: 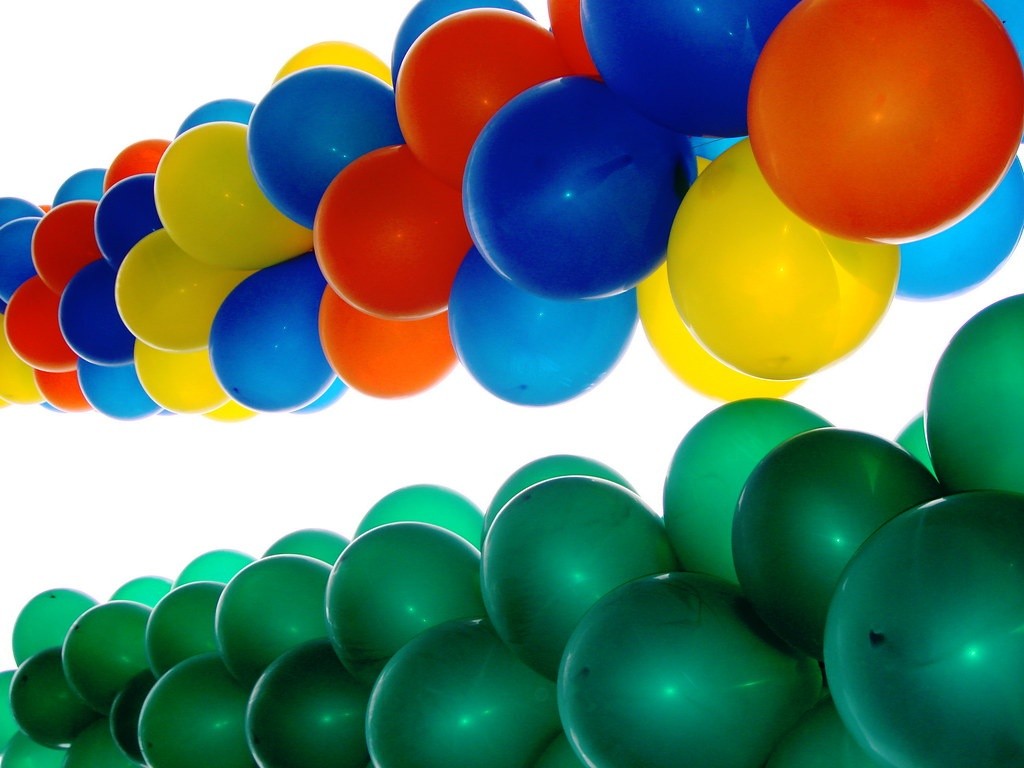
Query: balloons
[0,1,1024,767]
